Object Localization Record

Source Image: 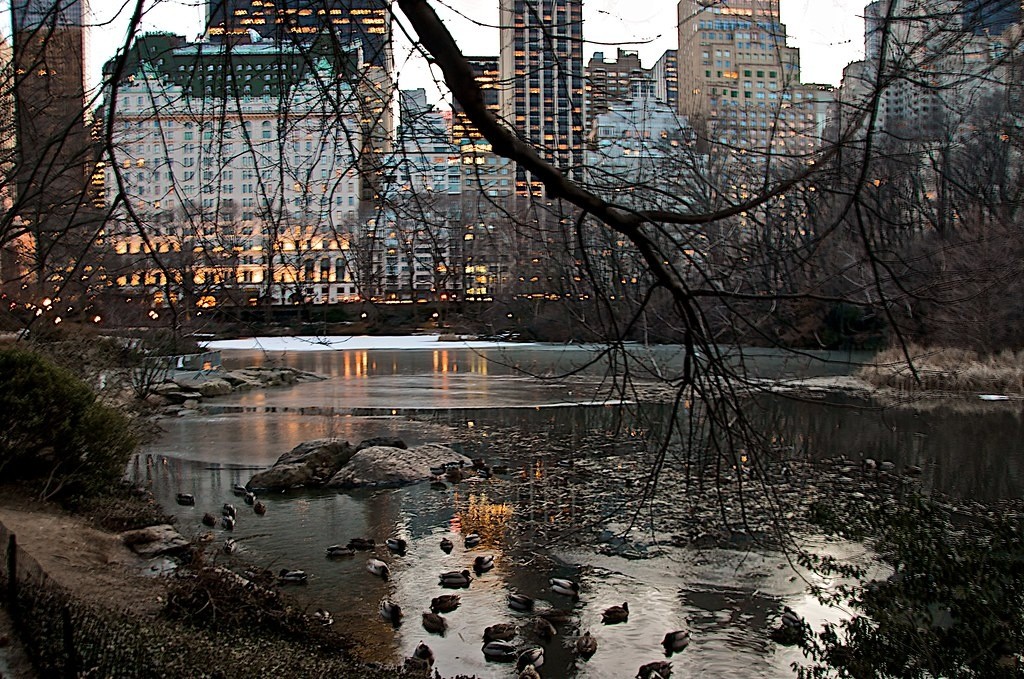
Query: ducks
[312,607,335,627]
[439,536,454,550]
[481,592,574,679]
[900,462,923,476]
[198,481,266,556]
[322,535,407,578]
[242,563,309,583]
[420,593,462,635]
[414,639,435,668]
[600,601,629,624]
[472,554,495,571]
[660,629,694,653]
[463,528,483,545]
[429,455,513,484]
[377,594,405,622]
[576,630,599,655]
[546,576,581,597]
[633,660,674,679]
[174,491,195,505]
[437,568,475,588]
[781,604,805,637]
